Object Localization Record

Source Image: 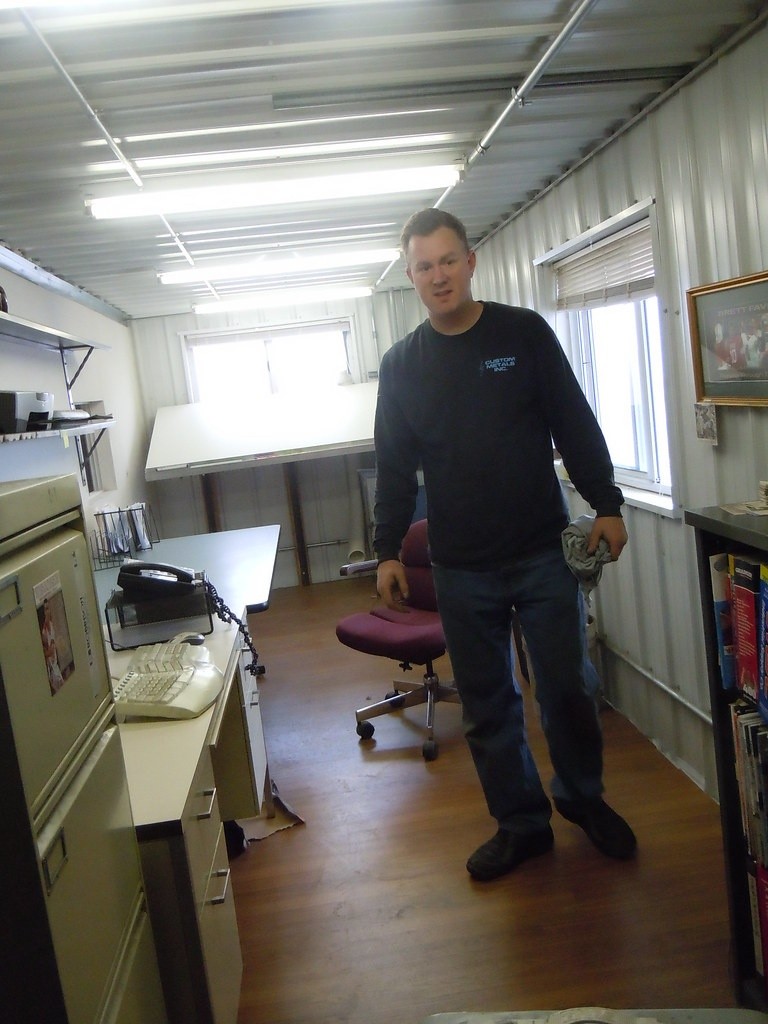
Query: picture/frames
[686,270,768,409]
[357,467,427,560]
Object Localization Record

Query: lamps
[84,148,468,220]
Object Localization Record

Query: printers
[0,390,54,434]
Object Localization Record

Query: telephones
[116,563,196,601]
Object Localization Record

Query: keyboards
[113,644,223,719]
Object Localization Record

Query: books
[708,552,768,980]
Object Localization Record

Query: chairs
[335,519,462,762]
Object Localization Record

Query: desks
[92,525,282,614]
[103,606,248,841]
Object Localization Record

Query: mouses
[168,632,205,645]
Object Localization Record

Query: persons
[41,599,65,692]
[372,209,637,880]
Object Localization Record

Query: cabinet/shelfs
[684,501,768,1015]
[1,311,117,493]
[210,617,268,822]
[138,746,243,1024]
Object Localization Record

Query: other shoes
[466,821,554,879]
[554,793,637,861]
